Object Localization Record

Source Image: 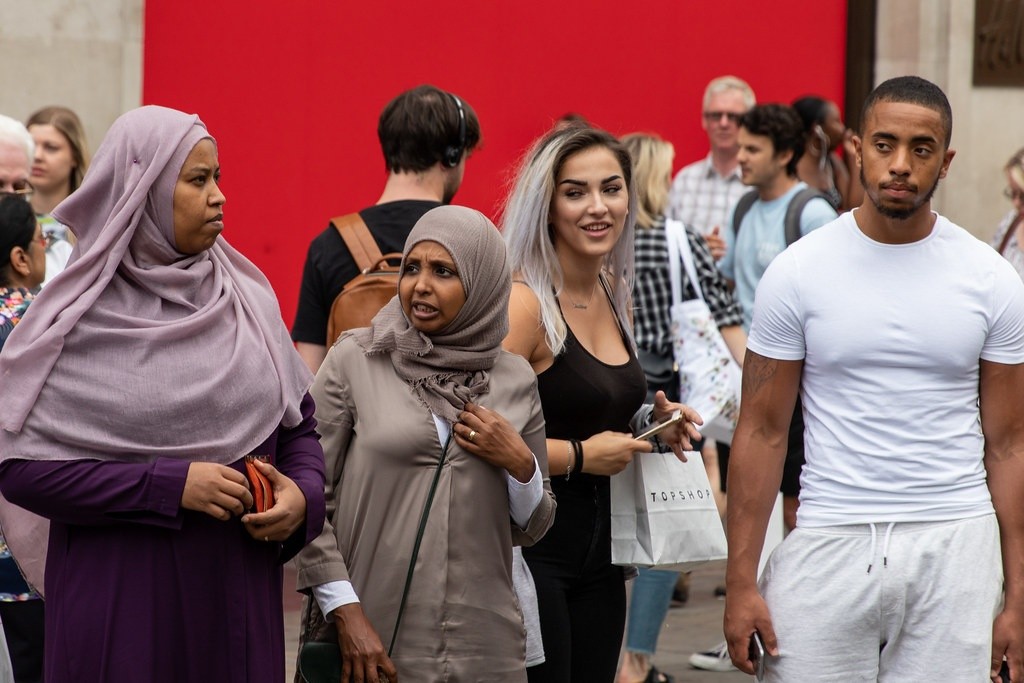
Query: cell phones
[631,409,684,441]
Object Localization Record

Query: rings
[265,536,270,542]
[468,431,475,440]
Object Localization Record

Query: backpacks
[326,212,403,354]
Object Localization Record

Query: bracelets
[566,439,584,481]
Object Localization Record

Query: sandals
[644,665,674,683]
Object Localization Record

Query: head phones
[815,125,822,137]
[442,92,465,168]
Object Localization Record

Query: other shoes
[671,582,689,605]
[714,585,725,601]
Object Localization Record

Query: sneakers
[689,640,740,671]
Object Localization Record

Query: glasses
[30,234,45,247]
[705,112,740,121]
[0,179,35,195]
[1004,187,1024,200]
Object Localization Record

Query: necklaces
[564,283,596,309]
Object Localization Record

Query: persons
[294,205,557,683]
[723,76,1024,683]
[618,76,864,683]
[501,126,703,683]
[988,148,1024,284]
[0,107,90,683]
[288,84,484,376]
[0,104,326,683]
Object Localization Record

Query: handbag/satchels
[298,641,355,683]
[665,217,744,446]
[610,418,731,566]
